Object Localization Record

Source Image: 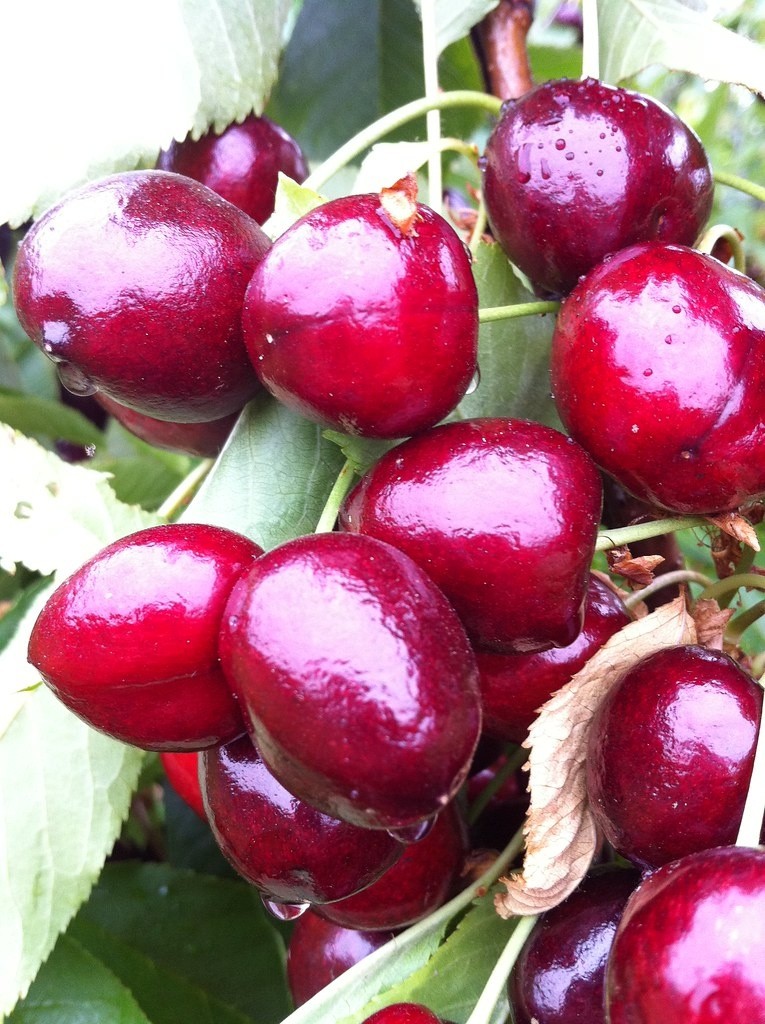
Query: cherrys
[10,77,765,1024]
